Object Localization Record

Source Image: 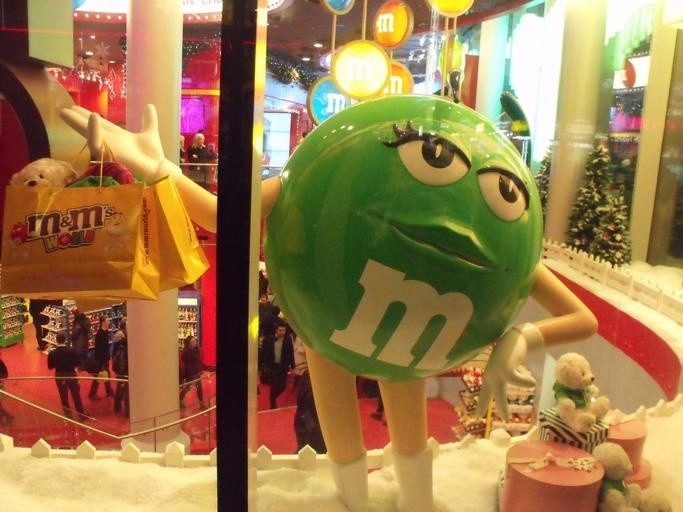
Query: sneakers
[65,390,129,420]
[180,398,185,408]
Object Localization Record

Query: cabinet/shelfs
[177,298,200,352]
[451,370,532,440]
[0,295,24,348]
[38,303,123,355]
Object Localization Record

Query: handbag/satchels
[0,142,211,301]
[97,371,108,382]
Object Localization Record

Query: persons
[110,318,129,417]
[88,316,113,399]
[187,134,208,183]
[0,359,15,422]
[47,334,90,420]
[179,336,208,412]
[70,309,90,372]
[29,300,49,351]
[259,271,327,454]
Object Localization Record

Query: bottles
[609,95,642,132]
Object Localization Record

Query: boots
[199,401,209,410]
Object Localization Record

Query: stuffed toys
[591,442,642,512]
[10,157,75,187]
[552,353,610,435]
